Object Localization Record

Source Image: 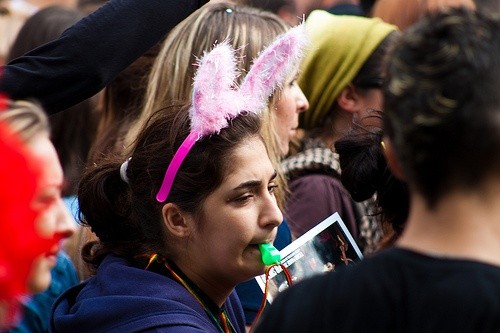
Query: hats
[295,9,397,131]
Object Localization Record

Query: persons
[120,0,309,207]
[279,10,401,258]
[0,100,76,333]
[0,1,211,117]
[8,249,79,333]
[258,0,500,333]
[4,5,102,167]
[85,45,157,174]
[47,104,284,333]
